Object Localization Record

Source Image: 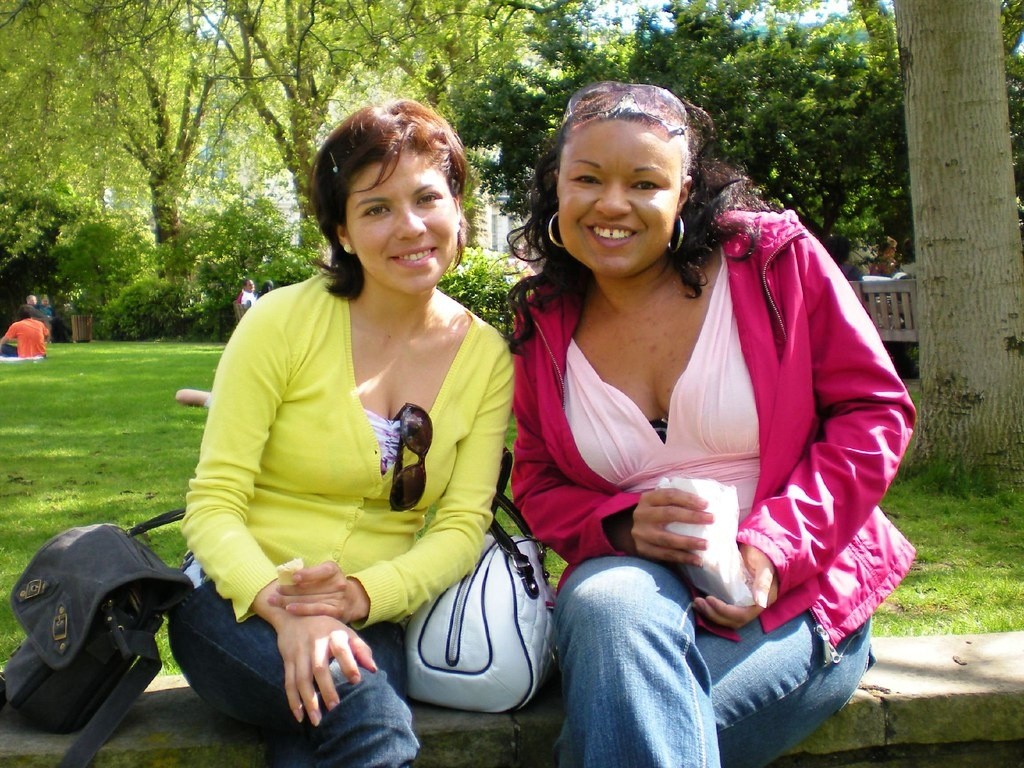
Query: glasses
[568,81,689,132]
[385,402,434,514]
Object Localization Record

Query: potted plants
[69,292,96,342]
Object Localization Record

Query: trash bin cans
[71,315,93,341]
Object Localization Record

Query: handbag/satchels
[406,447,555,713]
[1,508,197,733]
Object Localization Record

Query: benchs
[846,279,922,344]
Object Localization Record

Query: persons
[509,83,920,768]
[258,280,273,300]
[0,304,50,358]
[237,279,256,306]
[871,236,913,324]
[165,96,516,768]
